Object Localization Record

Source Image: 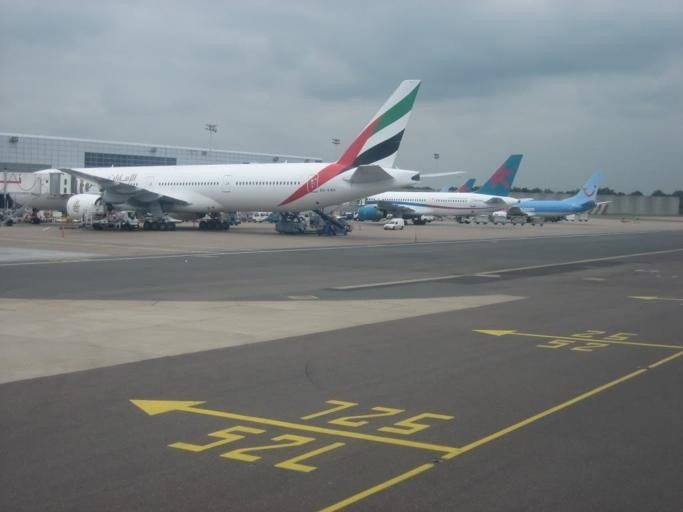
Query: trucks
[252,213,273,224]
[383,218,406,231]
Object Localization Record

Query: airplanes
[5,79,473,236]
[481,169,611,225]
[341,154,536,224]
